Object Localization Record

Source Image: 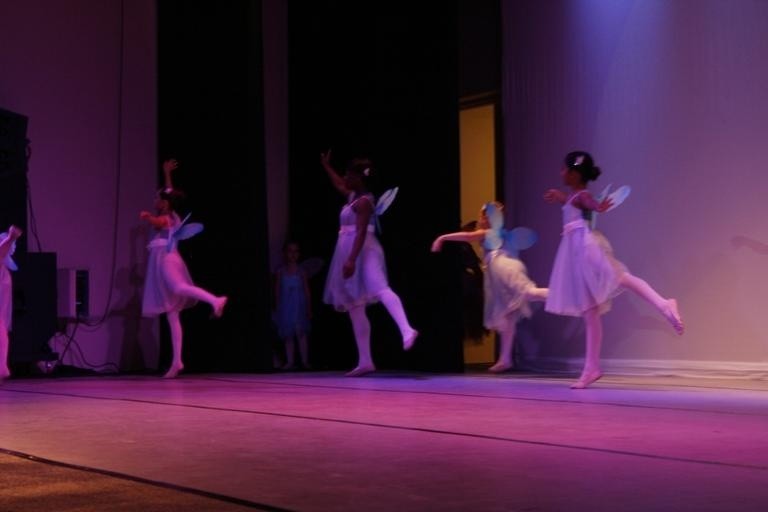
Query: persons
[431,201,551,373]
[272,238,314,373]
[138,158,227,379]
[0,225,21,376]
[320,149,419,377]
[543,151,684,389]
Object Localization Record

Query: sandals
[160,363,188,379]
[402,329,419,351]
[568,369,604,391]
[665,297,685,336]
[213,295,228,317]
[344,363,376,378]
[489,358,516,373]
